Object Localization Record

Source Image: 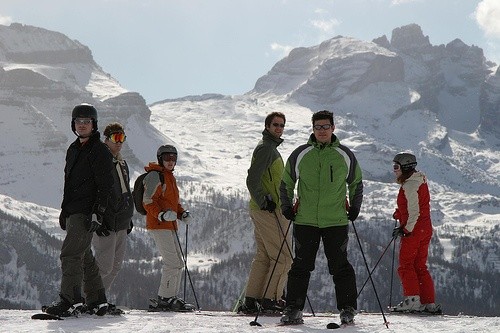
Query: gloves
[181,209,193,225]
[59,210,66,231]
[157,210,177,222]
[283,207,296,221]
[261,200,277,213]
[347,207,360,220]
[127,220,134,234]
[392,227,407,238]
[88,212,111,237]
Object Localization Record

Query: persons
[81,124,133,314]
[277,110,363,329]
[388,152,442,315]
[143,144,197,312]
[238,112,294,317]
[32,103,113,320]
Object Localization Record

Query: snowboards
[28,299,125,320]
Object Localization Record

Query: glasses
[271,122,285,128]
[107,133,126,144]
[162,153,177,162]
[393,164,401,172]
[73,119,93,125]
[314,124,333,130]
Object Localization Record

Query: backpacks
[132,169,165,214]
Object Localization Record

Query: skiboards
[197,296,339,318]
[248,307,355,329]
[362,293,480,318]
[124,295,235,313]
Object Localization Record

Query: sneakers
[241,295,304,325]
[387,295,422,312]
[156,294,195,310]
[339,310,353,325]
[44,295,124,316]
[421,302,438,312]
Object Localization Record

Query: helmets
[104,123,126,143]
[393,152,418,167]
[156,144,177,165]
[71,103,98,131]
